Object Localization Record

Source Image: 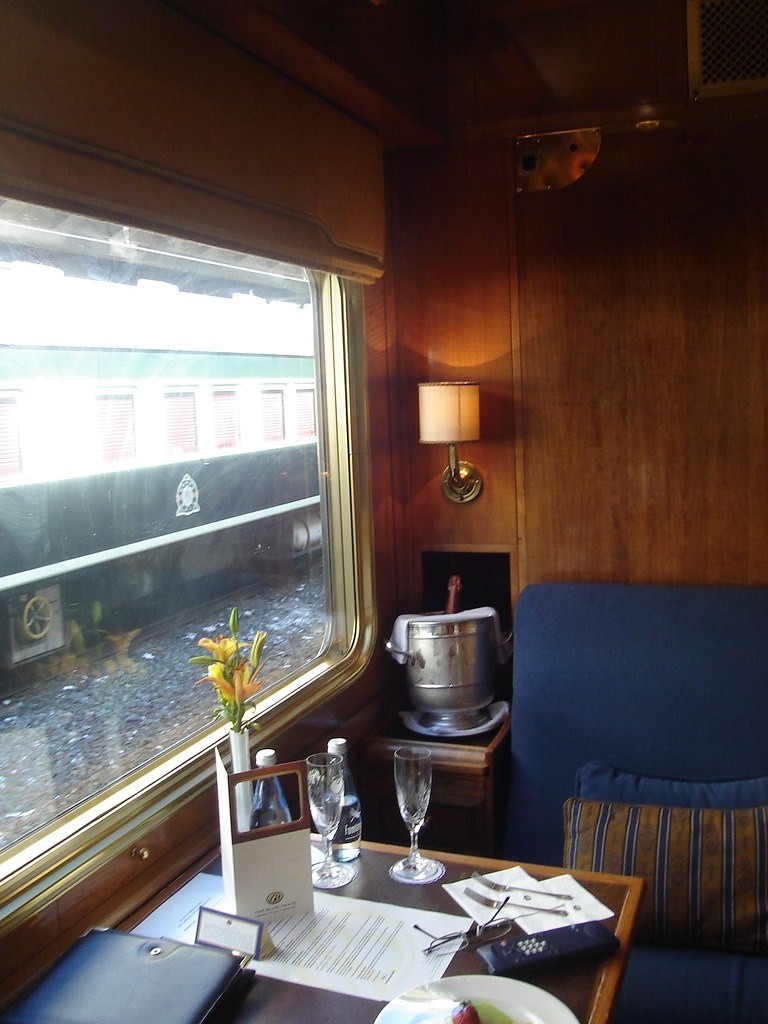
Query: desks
[117,834,645,1024]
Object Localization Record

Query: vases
[228,729,255,834]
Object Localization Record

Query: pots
[384,616,512,730]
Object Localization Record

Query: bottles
[327,738,362,862]
[249,748,292,831]
[444,575,463,614]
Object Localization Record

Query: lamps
[418,382,483,503]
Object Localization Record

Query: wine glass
[389,746,443,882]
[306,753,355,889]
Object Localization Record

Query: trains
[0,338,321,695]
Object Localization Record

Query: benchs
[502,581,768,1024]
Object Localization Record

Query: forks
[471,871,573,900]
[462,886,567,916]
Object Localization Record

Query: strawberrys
[451,1001,480,1024]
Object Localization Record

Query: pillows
[561,765,768,956]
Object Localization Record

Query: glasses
[413,897,516,959]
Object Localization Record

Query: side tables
[366,700,513,859]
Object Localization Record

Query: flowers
[187,606,268,730]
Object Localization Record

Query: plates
[373,974,580,1024]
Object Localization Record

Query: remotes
[475,920,619,979]
